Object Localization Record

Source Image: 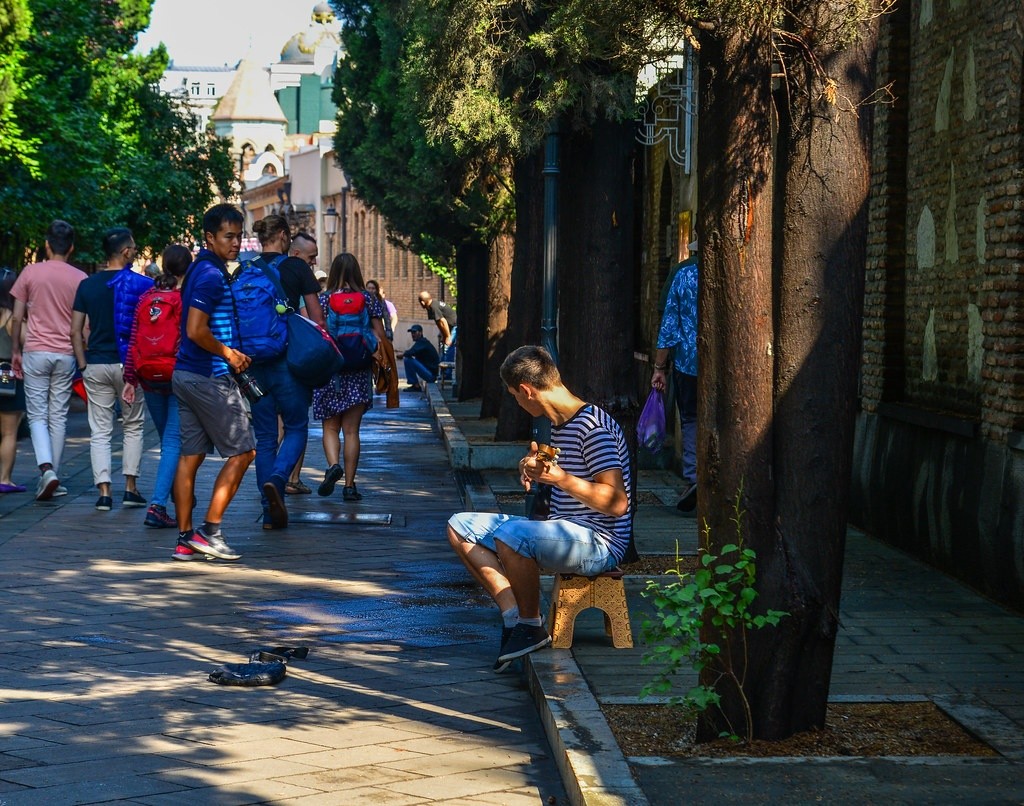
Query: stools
[547,564,634,648]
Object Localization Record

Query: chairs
[438,362,456,391]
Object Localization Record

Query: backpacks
[133,292,182,381]
[327,292,371,367]
[228,254,293,361]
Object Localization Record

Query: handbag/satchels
[0,362,17,396]
[285,306,343,387]
[636,387,666,456]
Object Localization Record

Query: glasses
[3,267,12,280]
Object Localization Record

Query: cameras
[227,365,264,404]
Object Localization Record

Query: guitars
[525,444,561,522]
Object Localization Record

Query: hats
[407,325,422,332]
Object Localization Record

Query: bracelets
[78,366,86,372]
[654,363,666,370]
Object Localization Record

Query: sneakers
[143,504,179,528]
[493,612,546,673]
[186,529,243,560]
[171,533,216,560]
[498,623,553,661]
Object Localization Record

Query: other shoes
[122,491,147,507]
[678,483,699,513]
[319,464,343,497]
[36,469,59,499]
[286,481,312,495]
[263,511,272,530]
[52,485,68,496]
[0,484,26,493]
[95,496,112,511]
[263,475,287,527]
[343,482,362,499]
[404,386,422,392]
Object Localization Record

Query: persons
[0,266,27,492]
[8,219,91,501]
[397,325,438,392]
[311,253,399,499]
[172,203,256,561]
[419,292,458,384]
[121,246,196,529]
[379,287,398,334]
[447,345,632,674]
[658,239,698,321]
[70,228,147,510]
[314,270,329,296]
[231,215,327,529]
[114,398,124,422]
[273,233,318,494]
[145,264,161,280]
[651,264,699,511]
[367,280,393,342]
